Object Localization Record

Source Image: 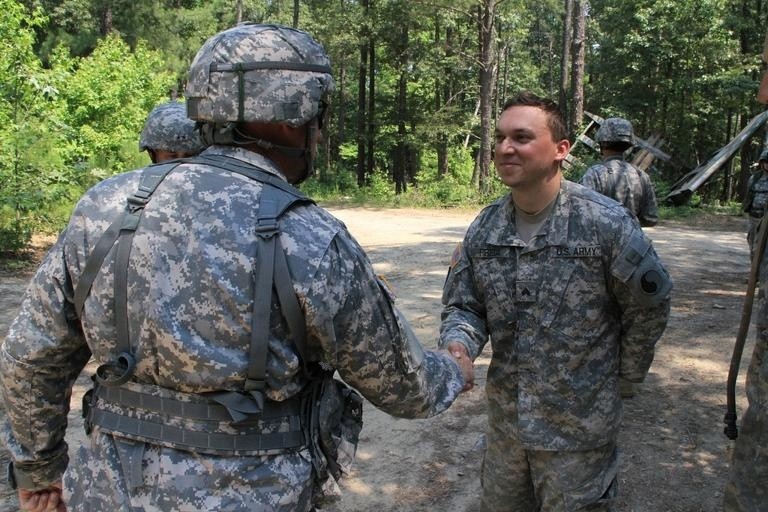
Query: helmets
[186,23,337,130]
[139,100,204,155]
[595,118,637,148]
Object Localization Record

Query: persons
[721,43,768,511]
[582,117,660,234]
[436,94,669,512]
[138,102,203,167]
[0,25,475,512]
